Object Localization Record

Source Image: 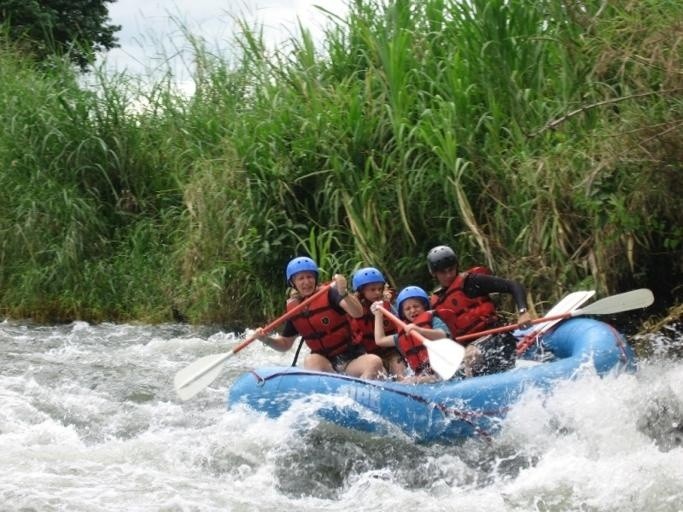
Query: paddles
[455,288,655,343]
[513,291,598,355]
[174,280,337,402]
[375,302,465,382]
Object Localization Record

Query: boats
[228,318,636,443]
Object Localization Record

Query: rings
[523,324,529,329]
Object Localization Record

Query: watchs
[519,308,527,314]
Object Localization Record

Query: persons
[369,286,466,383]
[425,245,532,377]
[348,268,406,382]
[254,257,382,383]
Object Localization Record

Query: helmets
[286,256,319,286]
[353,267,385,291]
[427,244,457,274]
[397,286,429,320]
